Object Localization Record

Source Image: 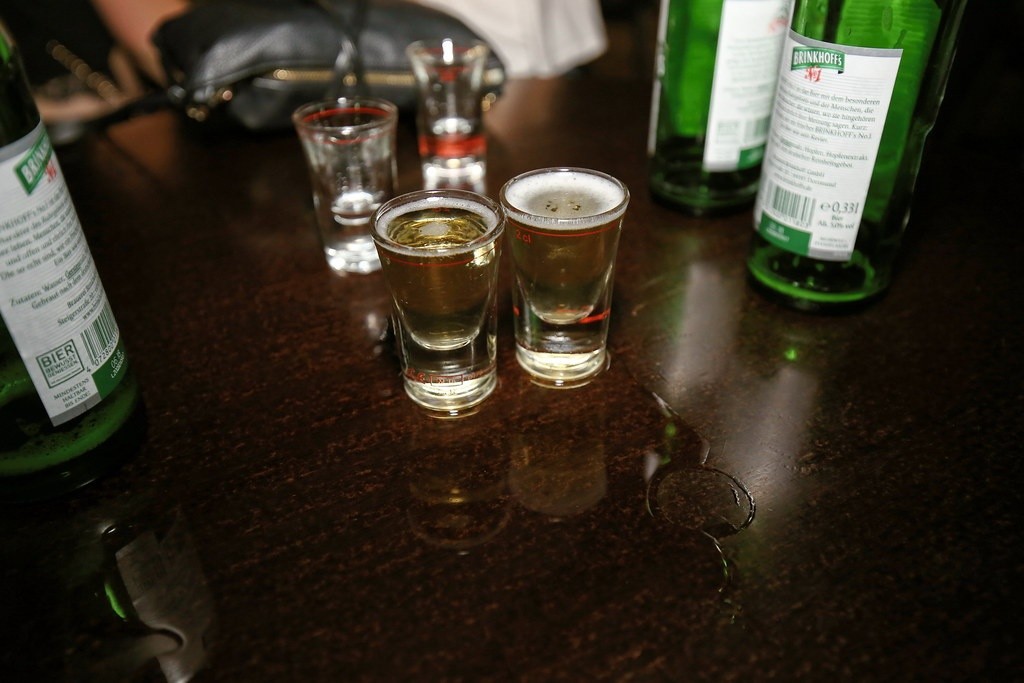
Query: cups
[292,96,399,278]
[369,189,506,419]
[409,39,490,160]
[500,166,630,389]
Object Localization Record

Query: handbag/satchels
[147,0,509,155]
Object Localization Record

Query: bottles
[748,0,968,314]
[0,21,151,503]
[645,0,792,216]
